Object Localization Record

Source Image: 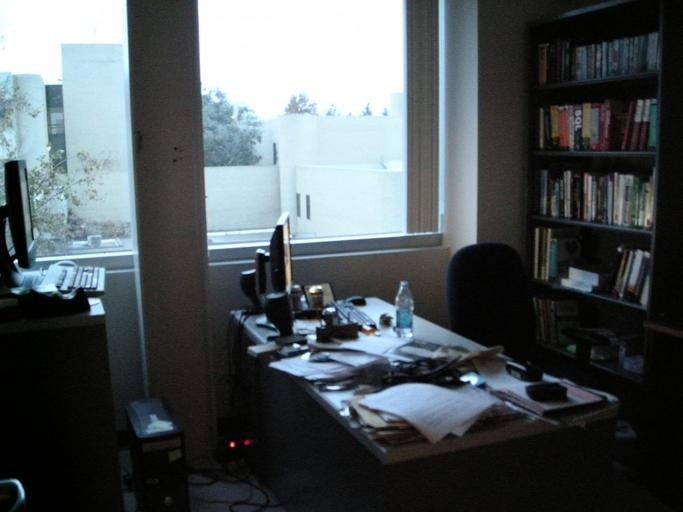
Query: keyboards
[326,300,376,327]
[57,264,107,296]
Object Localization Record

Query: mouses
[346,296,366,305]
[57,261,75,266]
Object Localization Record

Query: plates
[72,238,123,250]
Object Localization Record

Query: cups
[86,235,101,248]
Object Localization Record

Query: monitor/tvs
[0,160,36,299]
[255,211,293,332]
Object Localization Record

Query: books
[533,298,645,371]
[532,31,659,86]
[538,98,657,153]
[540,166,654,230]
[498,377,618,426]
[535,228,649,306]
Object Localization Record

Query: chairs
[443,242,539,368]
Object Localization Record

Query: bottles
[291,284,325,310]
[394,281,413,339]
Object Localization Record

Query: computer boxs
[125,398,190,512]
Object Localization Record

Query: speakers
[240,270,265,316]
[265,293,306,346]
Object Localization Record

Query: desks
[226,293,620,512]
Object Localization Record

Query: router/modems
[217,415,256,459]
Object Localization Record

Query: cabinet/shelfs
[1,296,125,512]
[524,0,682,387]
